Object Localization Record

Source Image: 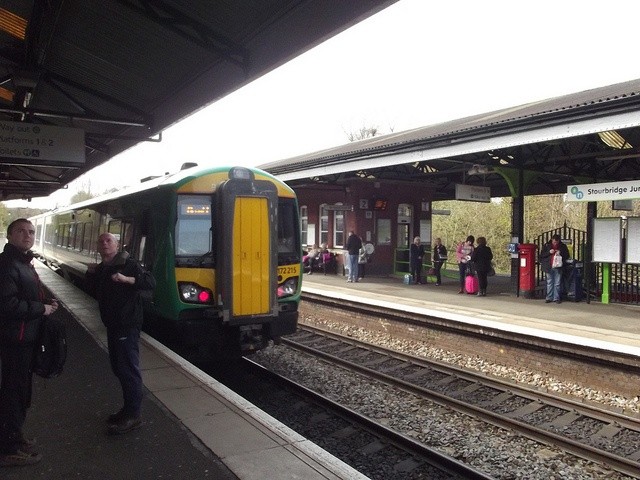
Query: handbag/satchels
[302,255,309,263]
[552,252,563,268]
[438,253,447,260]
[487,268,495,276]
[404,273,413,285]
[323,253,331,264]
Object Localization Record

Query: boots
[482,289,486,296]
[477,289,483,297]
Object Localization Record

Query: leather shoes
[108,412,123,423]
[109,417,143,433]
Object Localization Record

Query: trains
[26,162,303,356]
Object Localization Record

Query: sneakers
[19,438,37,451]
[355,279,358,281]
[546,300,552,303]
[0,448,41,466]
[307,271,312,274]
[346,279,352,282]
[436,284,441,286]
[554,300,559,304]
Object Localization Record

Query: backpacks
[30,316,67,379]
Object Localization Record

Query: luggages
[567,267,583,302]
[465,262,478,295]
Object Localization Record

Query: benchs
[305,253,336,275]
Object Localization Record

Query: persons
[0,219,59,466]
[357,236,365,280]
[540,234,569,304]
[308,243,328,275]
[434,238,447,285]
[411,237,425,283]
[457,235,475,293]
[76,232,146,433]
[346,231,361,282]
[473,237,493,296]
[303,244,319,275]
